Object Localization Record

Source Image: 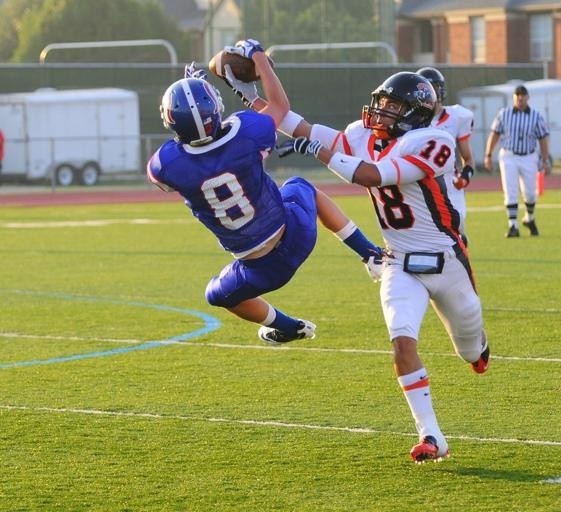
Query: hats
[514,85,530,97]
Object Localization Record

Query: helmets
[414,67,448,102]
[159,77,227,149]
[370,69,439,141]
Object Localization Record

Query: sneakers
[523,217,540,237]
[363,244,389,282]
[257,316,319,345]
[471,344,491,374]
[506,224,521,239]
[410,434,451,466]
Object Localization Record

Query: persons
[483,84,551,238]
[415,67,476,248]
[215,65,490,464]
[147,39,387,343]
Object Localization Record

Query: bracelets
[484,154,491,158]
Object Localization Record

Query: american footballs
[208,50,275,82]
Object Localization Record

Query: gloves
[222,62,261,109]
[273,136,323,160]
[452,165,475,191]
[223,38,265,60]
[183,60,208,81]
